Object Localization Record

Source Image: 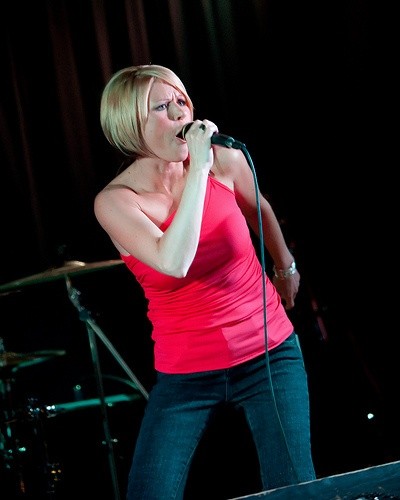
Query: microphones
[183,122,246,149]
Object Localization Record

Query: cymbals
[0,259,124,290]
[0,350,66,373]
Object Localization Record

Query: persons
[94,64,316,500]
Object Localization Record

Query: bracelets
[273,260,297,281]
